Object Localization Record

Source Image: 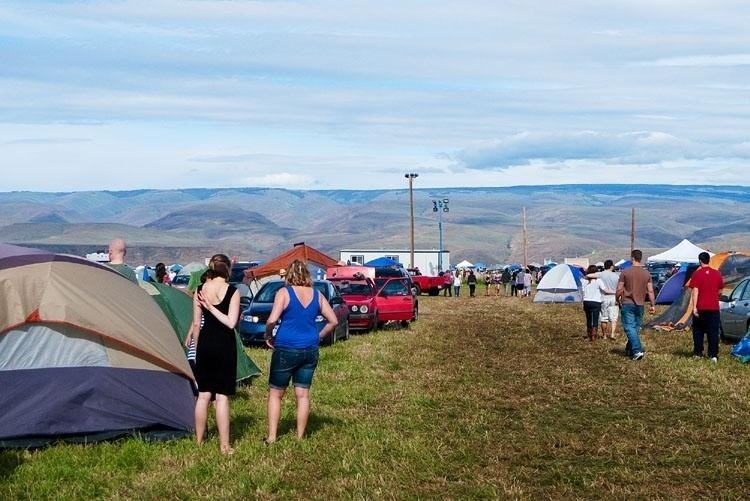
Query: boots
[586,326,598,342]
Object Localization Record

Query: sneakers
[692,354,704,360]
[709,357,718,363]
[625,349,645,362]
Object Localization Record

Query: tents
[595,259,633,272]
[135,259,209,293]
[650,251,750,337]
[1,245,209,459]
[143,277,263,394]
[532,263,589,303]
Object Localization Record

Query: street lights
[431,198,450,273]
[405,173,419,268]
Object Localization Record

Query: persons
[583,260,620,340]
[688,252,725,363]
[103,239,139,289]
[193,254,242,458]
[182,269,213,380]
[615,249,657,362]
[439,259,556,303]
[579,264,617,341]
[261,258,339,447]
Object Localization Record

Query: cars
[485,269,504,283]
[718,277,750,345]
[165,260,447,350]
[645,263,677,301]
